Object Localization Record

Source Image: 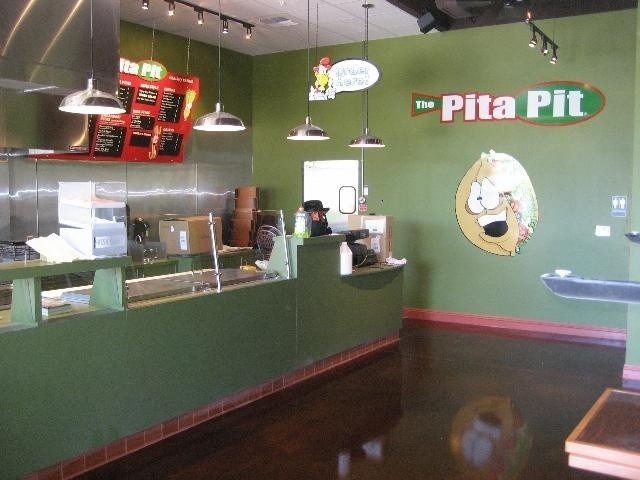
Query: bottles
[294,206,311,239]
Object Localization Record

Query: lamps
[525,19,560,65]
[192,0,247,134]
[347,5,387,149]
[57,0,128,115]
[141,0,254,41]
[286,0,333,142]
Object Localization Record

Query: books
[567,455,640,480]
[565,388,640,469]
[41,297,71,317]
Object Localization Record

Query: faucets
[135,234,142,244]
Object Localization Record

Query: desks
[563,386,640,479]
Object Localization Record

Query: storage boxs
[157,214,224,257]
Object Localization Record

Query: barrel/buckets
[291,205,312,239]
